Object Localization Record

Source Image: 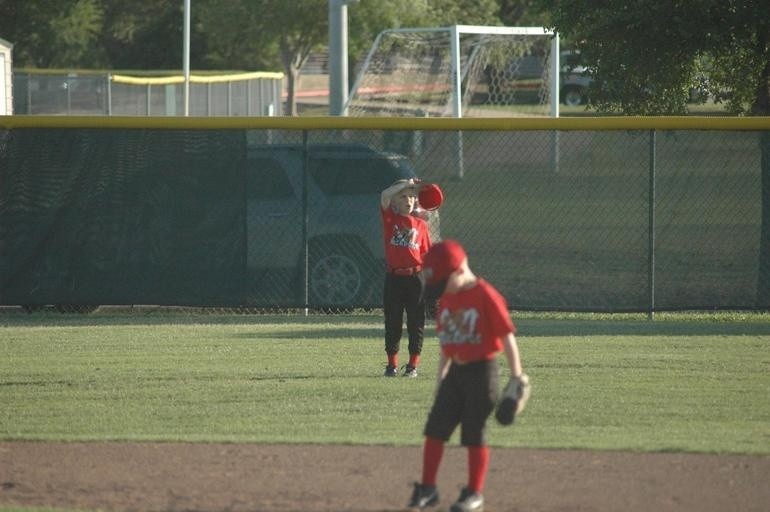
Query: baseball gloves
[497,374,530,424]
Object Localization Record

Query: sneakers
[451,488,483,512]
[384,364,417,376]
[407,482,439,512]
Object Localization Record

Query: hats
[420,240,466,300]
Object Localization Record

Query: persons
[407,239,530,512]
[380,174,434,377]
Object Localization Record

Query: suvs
[558,47,709,105]
[18,138,441,319]
